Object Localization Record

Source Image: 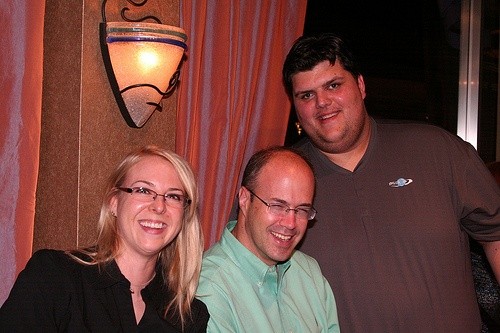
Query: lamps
[100,0,187,128]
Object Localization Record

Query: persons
[282,34,500,333]
[194,146,341,333]
[0,145,211,333]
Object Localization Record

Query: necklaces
[129,271,156,294]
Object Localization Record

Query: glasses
[115,184,191,209]
[236,186,317,221]
